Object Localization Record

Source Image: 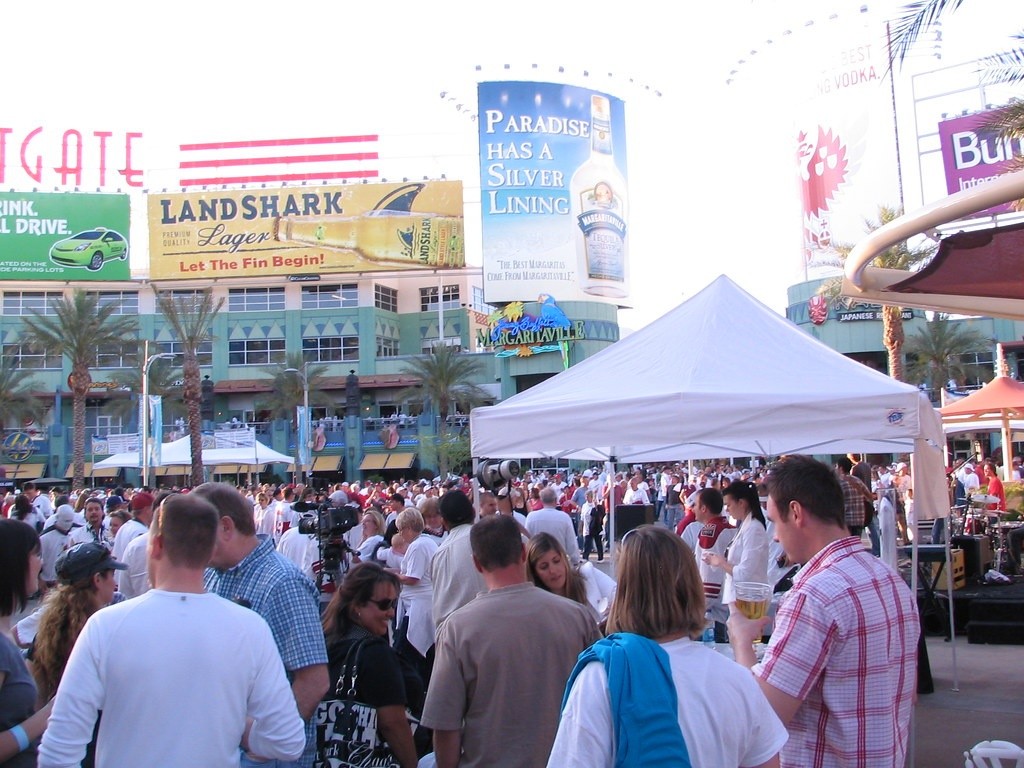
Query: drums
[951,514,1024,537]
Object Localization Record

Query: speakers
[950,535,992,581]
[614,504,655,541]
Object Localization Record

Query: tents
[98,274,1024,691]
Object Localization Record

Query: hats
[965,464,976,472]
[106,497,120,507]
[55,541,129,577]
[390,493,404,503]
[423,485,434,492]
[895,463,907,472]
[437,489,472,522]
[132,493,154,509]
[499,487,522,499]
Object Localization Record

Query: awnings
[139,466,192,476]
[1,464,47,478]
[285,455,345,472]
[213,465,268,474]
[358,452,417,468]
[65,462,121,477]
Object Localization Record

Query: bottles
[703,609,715,649]
[569,95,627,299]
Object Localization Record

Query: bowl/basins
[383,567,402,574]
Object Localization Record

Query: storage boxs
[931,549,965,590]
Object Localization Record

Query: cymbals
[974,507,1012,517]
[972,494,1001,503]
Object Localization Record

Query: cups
[702,550,711,558]
[735,582,772,644]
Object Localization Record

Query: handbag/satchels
[317,637,419,768]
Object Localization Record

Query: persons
[0,413,1024,768]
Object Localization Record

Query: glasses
[367,597,398,610]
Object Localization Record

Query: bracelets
[10,724,28,753]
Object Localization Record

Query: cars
[51,228,127,271]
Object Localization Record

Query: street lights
[142,353,177,492]
[284,362,310,488]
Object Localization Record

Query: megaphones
[477,459,520,490]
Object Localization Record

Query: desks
[899,544,954,639]
